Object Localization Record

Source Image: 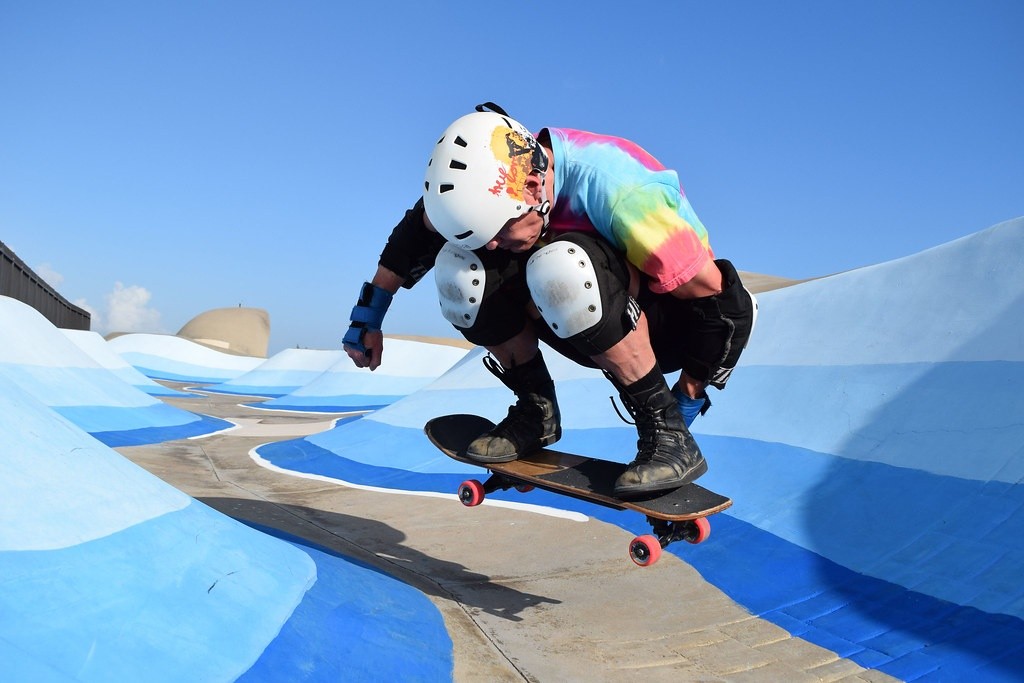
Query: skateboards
[425,410,736,568]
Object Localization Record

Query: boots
[601,369,708,500]
[466,351,561,462]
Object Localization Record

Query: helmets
[423,111,550,251]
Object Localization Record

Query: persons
[343,102,758,496]
